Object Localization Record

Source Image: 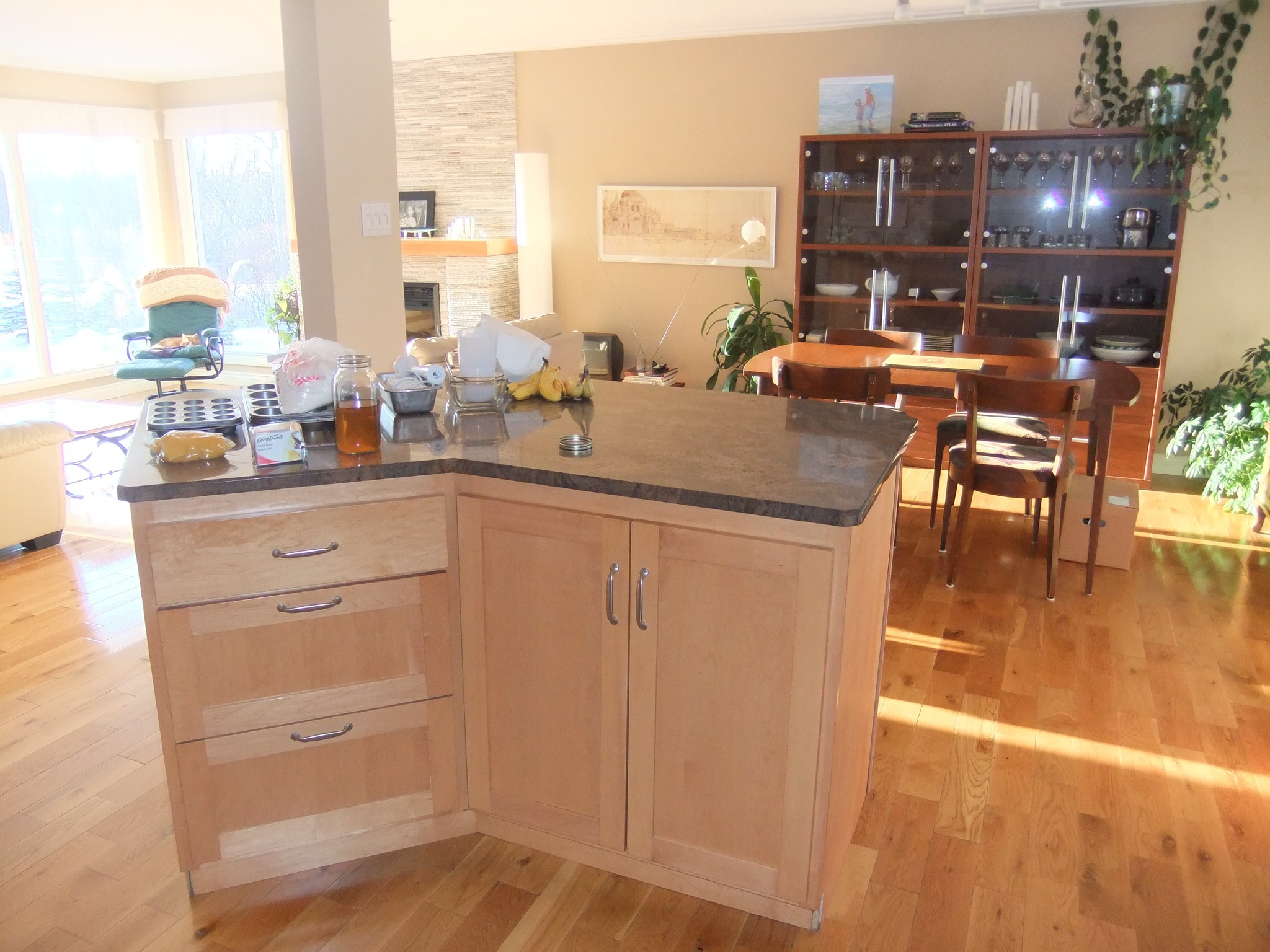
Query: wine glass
[856,144,1178,192]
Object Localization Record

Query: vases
[1067,70,1104,127]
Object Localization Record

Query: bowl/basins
[1036,332,1152,364]
[930,287,961,301]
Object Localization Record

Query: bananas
[506,357,595,402]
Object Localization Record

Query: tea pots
[865,266,902,298]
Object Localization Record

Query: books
[899,111,975,132]
[622,365,678,386]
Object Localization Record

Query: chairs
[939,371,1096,602]
[113,266,232,402]
[928,334,1058,529]
[771,358,891,407]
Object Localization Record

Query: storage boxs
[1057,472,1140,572]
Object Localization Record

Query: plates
[989,292,1040,305]
[815,283,858,295]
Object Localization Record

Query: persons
[862,86,876,128]
[400,201,416,228]
[412,200,426,228]
[854,98,863,128]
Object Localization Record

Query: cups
[635,356,645,372]
[331,355,382,455]
[810,170,851,190]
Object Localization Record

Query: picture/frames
[399,190,435,238]
[597,185,777,268]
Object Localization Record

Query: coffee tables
[0,398,142,499]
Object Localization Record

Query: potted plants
[1074,0,1258,215]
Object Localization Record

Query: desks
[743,342,1141,595]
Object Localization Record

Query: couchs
[0,420,76,551]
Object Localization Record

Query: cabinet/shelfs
[792,129,982,474]
[970,127,1198,489]
[453,457,911,921]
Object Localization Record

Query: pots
[1109,278,1156,307]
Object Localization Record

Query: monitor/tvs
[582,332,624,381]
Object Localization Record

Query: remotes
[652,363,667,369]
